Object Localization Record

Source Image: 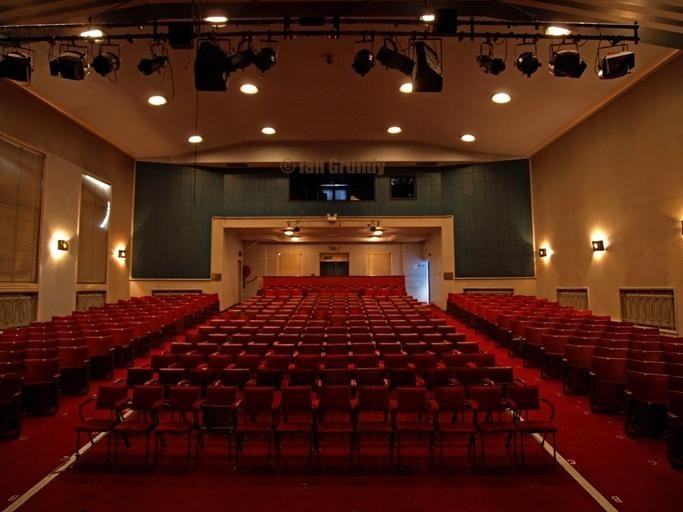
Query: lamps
[138,56,166,75]
[170,21,277,93]
[590,240,604,252]
[277,213,384,241]
[57,239,69,251]
[537,248,546,258]
[512,43,636,80]
[476,42,505,74]
[376,36,443,95]
[350,36,376,76]
[118,249,126,258]
[0,43,34,88]
[47,40,121,84]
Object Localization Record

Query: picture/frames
[386,173,417,202]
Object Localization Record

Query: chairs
[1,292,219,444]
[74,287,561,468]
[448,292,682,470]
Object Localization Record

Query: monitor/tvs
[390,176,418,200]
